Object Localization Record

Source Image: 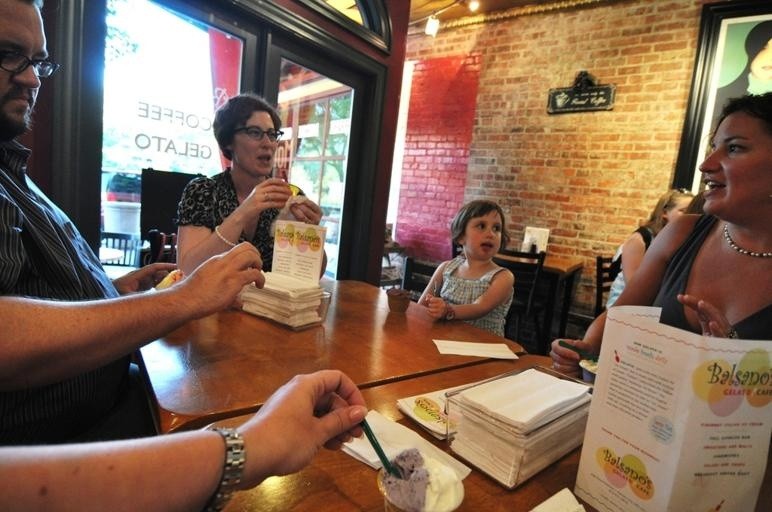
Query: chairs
[596,256,623,318]
[452,238,464,258]
[399,257,437,303]
[148,230,176,265]
[493,249,547,353]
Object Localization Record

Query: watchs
[444,300,455,322]
[201,426,248,511]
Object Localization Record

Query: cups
[376,461,465,512]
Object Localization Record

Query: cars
[105,171,145,204]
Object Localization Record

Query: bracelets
[214,226,238,248]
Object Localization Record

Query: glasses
[0,48,61,80]
[230,125,284,142]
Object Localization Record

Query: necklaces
[723,224,772,259]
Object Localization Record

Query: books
[271,219,326,286]
[519,226,550,252]
[394,383,459,439]
[573,306,772,512]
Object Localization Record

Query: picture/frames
[668,1,772,194]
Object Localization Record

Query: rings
[726,328,739,340]
[264,192,269,199]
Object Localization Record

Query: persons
[1,369,367,511]
[176,95,327,279]
[607,189,696,309]
[417,200,515,339]
[550,92,771,378]
[0,0,265,446]
[709,21,772,131]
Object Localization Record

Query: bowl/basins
[579,359,598,381]
[386,289,411,313]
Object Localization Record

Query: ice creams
[378,447,464,510]
[388,289,412,311]
[579,356,598,382]
[150,268,186,291]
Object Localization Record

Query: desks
[137,280,526,435]
[493,251,585,353]
[192,353,601,511]
